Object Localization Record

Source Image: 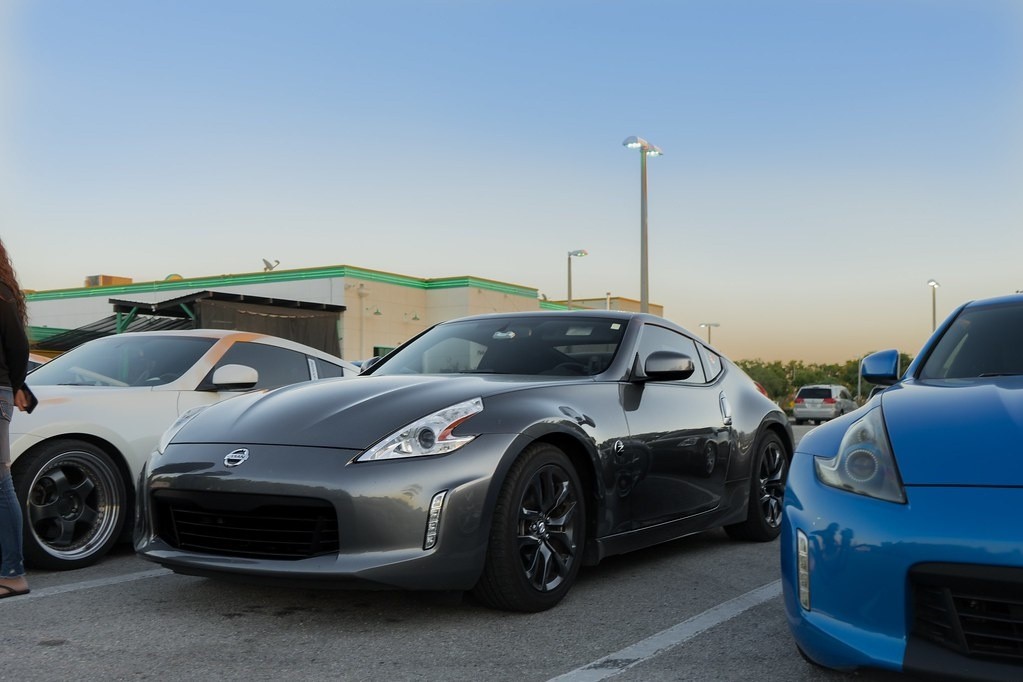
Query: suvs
[792,383,859,425]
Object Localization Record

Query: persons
[0,241,31,598]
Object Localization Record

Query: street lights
[699,321,721,345]
[564,249,589,309]
[622,136,664,310]
[927,280,940,334]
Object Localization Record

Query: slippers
[0,584,31,600]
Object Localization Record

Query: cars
[130,308,796,619]
[10,328,367,571]
[778,288,1023,682]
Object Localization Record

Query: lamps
[404,311,419,320]
[366,305,382,315]
